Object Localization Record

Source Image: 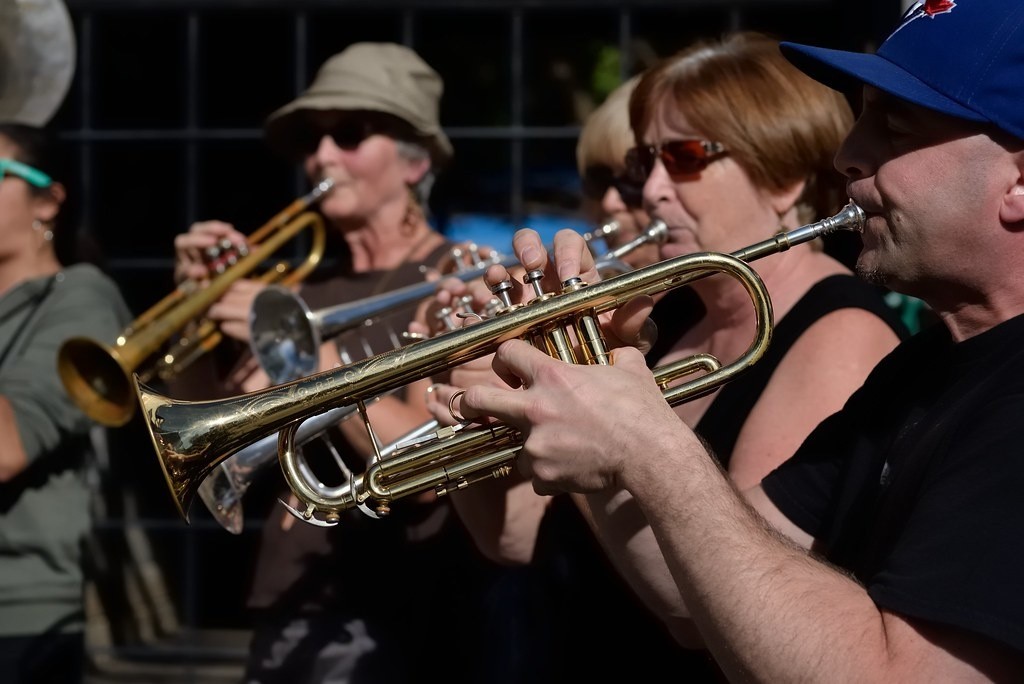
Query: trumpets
[54,171,339,428]
[130,194,866,528]
[246,211,626,387]
[196,214,670,535]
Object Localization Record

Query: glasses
[626,139,730,185]
[305,119,418,157]
[581,171,645,209]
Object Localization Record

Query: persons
[0,33,901,684]
[461,1,1024,684]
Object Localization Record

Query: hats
[778,0,1024,142]
[271,42,453,159]
